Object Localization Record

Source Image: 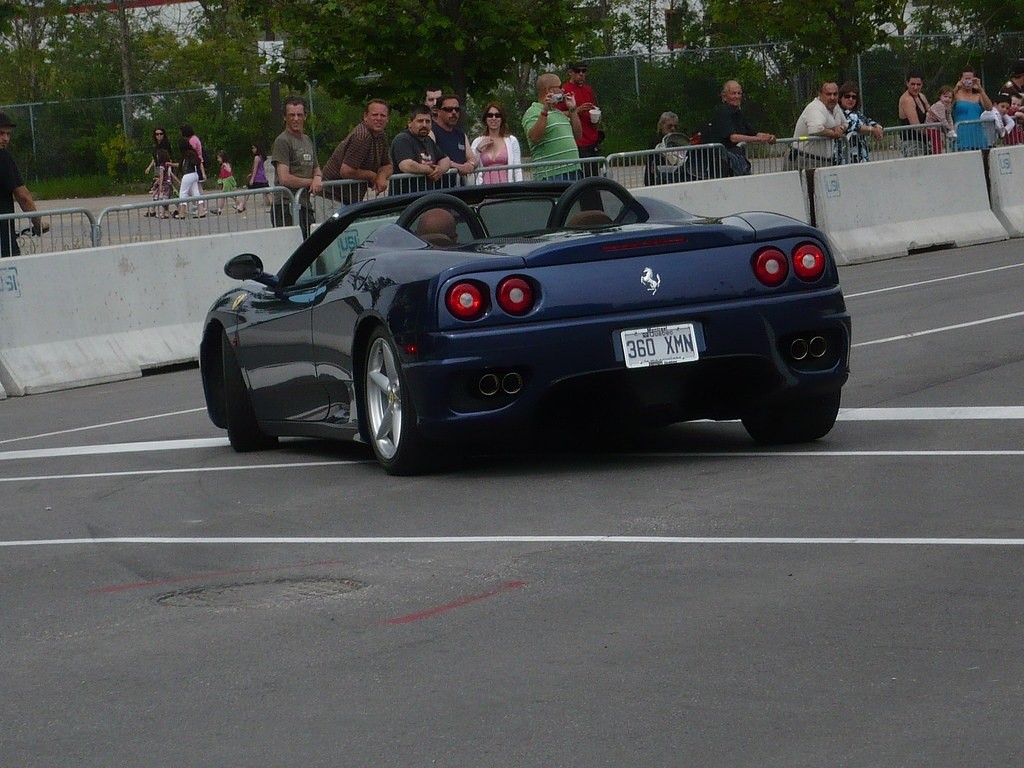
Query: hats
[0,111,16,128]
[569,60,587,69]
[1013,60,1024,74]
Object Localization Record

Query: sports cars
[196,175,854,477]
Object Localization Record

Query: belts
[579,139,601,151]
[788,144,831,162]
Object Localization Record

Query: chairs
[395,195,488,251]
[546,176,649,231]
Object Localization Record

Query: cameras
[551,94,563,104]
[965,81,972,86]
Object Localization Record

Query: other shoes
[143,203,272,219]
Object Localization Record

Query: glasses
[911,83,921,86]
[486,113,501,118]
[543,84,562,90]
[667,123,677,129]
[822,92,837,96]
[574,69,586,73]
[156,133,164,135]
[843,94,857,100]
[438,106,459,113]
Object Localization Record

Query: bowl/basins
[656,165,678,172]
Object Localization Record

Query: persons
[924,85,957,154]
[998,66,1024,145]
[641,112,691,188]
[522,74,583,182]
[471,103,524,185]
[417,207,458,244]
[784,82,849,170]
[424,86,446,123]
[143,124,208,219]
[556,60,604,181]
[269,96,323,241]
[319,98,393,205]
[387,105,450,194]
[210,150,239,215]
[428,94,478,188]
[234,142,272,212]
[704,79,776,178]
[898,74,933,157]
[830,81,884,165]
[950,66,993,152]
[0,111,50,258]
[980,94,1015,148]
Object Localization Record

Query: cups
[589,110,601,124]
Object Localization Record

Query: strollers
[649,131,711,185]
